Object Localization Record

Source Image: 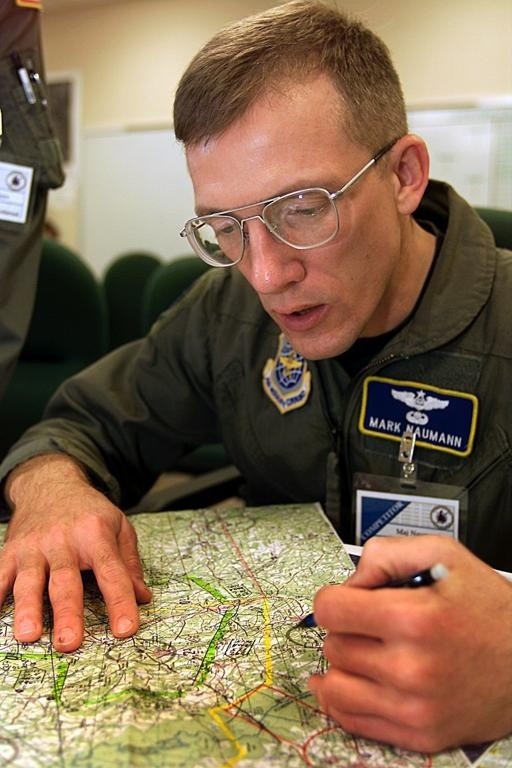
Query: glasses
[179,137,402,269]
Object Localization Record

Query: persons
[1,1,511,755]
[1,1,68,397]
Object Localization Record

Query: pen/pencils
[12,50,40,107]
[293,563,450,629]
[25,58,48,107]
